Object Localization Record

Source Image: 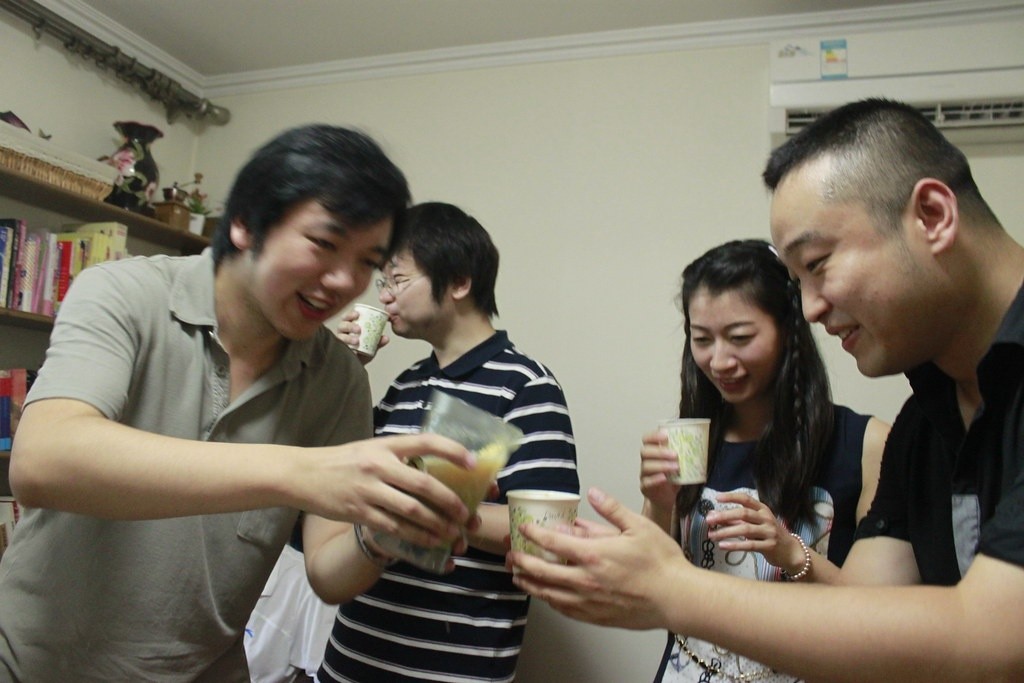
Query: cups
[658,417,710,484]
[371,389,524,574]
[505,489,582,574]
[347,302,390,357]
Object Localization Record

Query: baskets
[0,146,113,202]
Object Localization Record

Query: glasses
[376,272,426,296]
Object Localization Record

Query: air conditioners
[767,17,1024,154]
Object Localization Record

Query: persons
[0,124,500,683]
[305,203,580,683]
[634,240,891,683]
[504,94,1024,683]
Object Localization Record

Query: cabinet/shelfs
[0,145,213,551]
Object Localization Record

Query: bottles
[106,121,165,212]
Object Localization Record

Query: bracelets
[783,533,810,581]
[352,524,396,568]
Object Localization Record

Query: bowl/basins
[162,188,189,202]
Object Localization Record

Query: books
[1,220,130,319]
[1,369,42,496]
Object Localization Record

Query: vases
[189,213,207,236]
[104,120,164,213]
[187,189,213,215]
